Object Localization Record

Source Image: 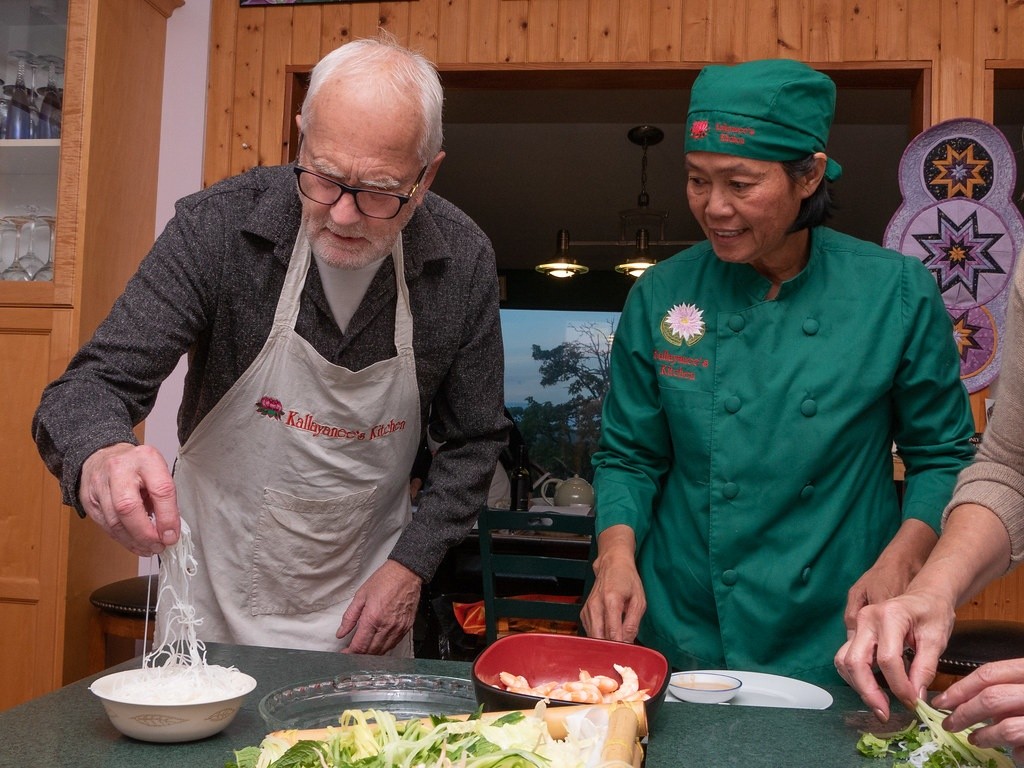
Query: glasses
[293,131,429,219]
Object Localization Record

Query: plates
[259,672,477,732]
[664,670,833,710]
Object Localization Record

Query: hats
[684,58,843,184]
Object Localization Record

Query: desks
[0,642,1024,768]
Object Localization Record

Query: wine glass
[0,50,64,139]
[0,216,55,281]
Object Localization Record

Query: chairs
[475,503,597,649]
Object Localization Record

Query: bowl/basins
[668,673,742,704]
[91,665,257,742]
[472,633,671,734]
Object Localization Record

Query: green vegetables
[856,699,1021,768]
[230,695,612,767]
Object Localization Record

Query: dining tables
[412,498,593,660]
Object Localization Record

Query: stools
[89,574,159,675]
[904,619,1024,675]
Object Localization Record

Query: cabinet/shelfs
[0,0,187,715]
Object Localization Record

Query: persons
[833,246,1024,766]
[581,57,978,688]
[30,39,503,660]
[405,408,512,532]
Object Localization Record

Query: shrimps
[491,664,652,705]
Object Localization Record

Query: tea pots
[541,474,594,509]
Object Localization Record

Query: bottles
[511,446,529,512]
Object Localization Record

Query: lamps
[535,126,703,279]
